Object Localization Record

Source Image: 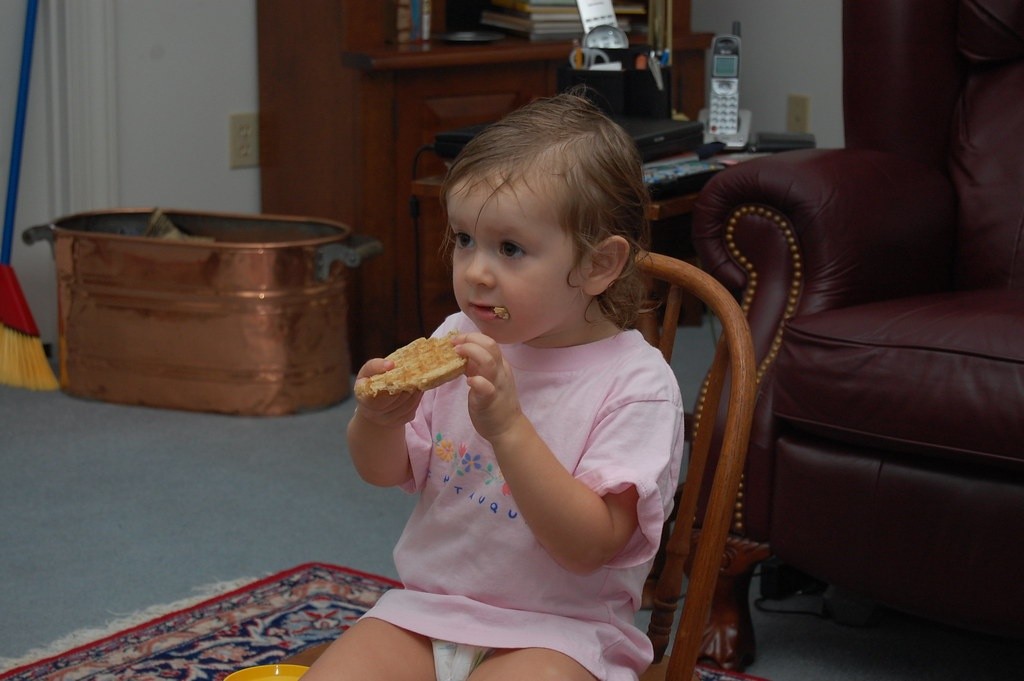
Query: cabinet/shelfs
[258,0,718,370]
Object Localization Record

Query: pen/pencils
[576,48,582,69]
[634,46,671,70]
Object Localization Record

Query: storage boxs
[24,207,351,417]
[557,65,672,119]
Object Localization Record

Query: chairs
[282,247,756,680]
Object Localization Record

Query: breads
[353,330,469,399]
[494,306,510,319]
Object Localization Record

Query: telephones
[695,19,754,158]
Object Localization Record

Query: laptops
[433,117,703,163]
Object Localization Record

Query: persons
[297,93,686,681]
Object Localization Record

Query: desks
[409,150,777,352]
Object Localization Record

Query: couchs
[691,0,1023,673]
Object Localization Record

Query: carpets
[1,561,770,681]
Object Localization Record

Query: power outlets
[788,94,810,135]
[230,112,259,167]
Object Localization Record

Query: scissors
[569,47,609,70]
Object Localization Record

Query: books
[397,0,431,43]
[749,132,815,151]
[480,0,645,40]
